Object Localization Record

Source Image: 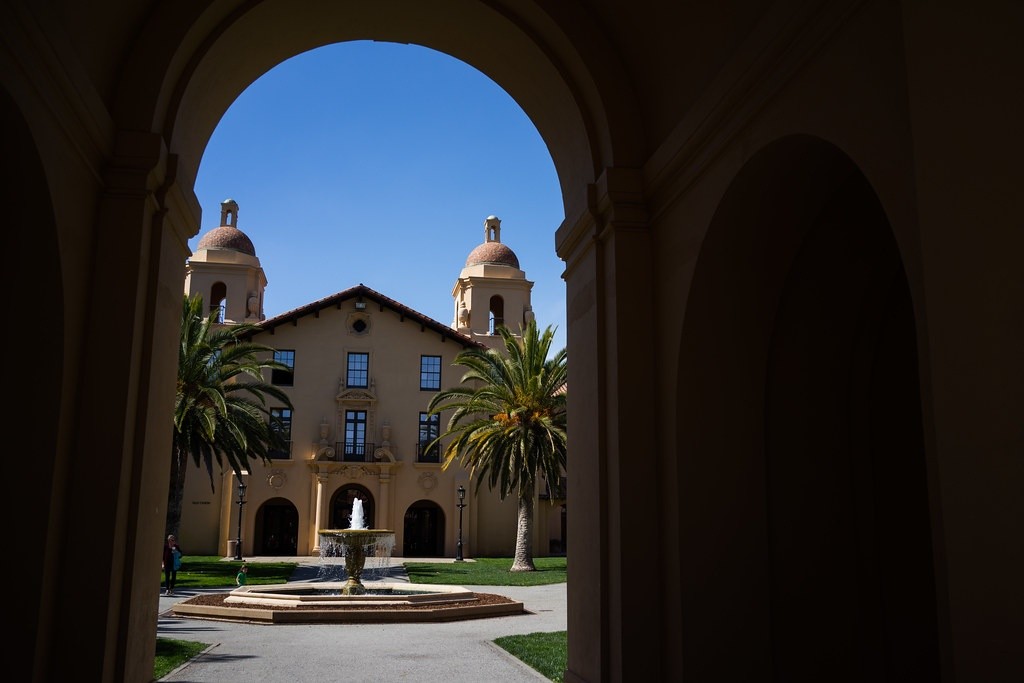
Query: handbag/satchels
[174,550,181,570]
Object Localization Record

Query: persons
[162,535,182,595]
[235,565,248,587]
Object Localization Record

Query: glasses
[171,539,176,541]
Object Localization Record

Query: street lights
[456,485,468,560]
[235,482,248,560]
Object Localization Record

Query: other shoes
[165,589,169,596]
[170,588,175,595]
